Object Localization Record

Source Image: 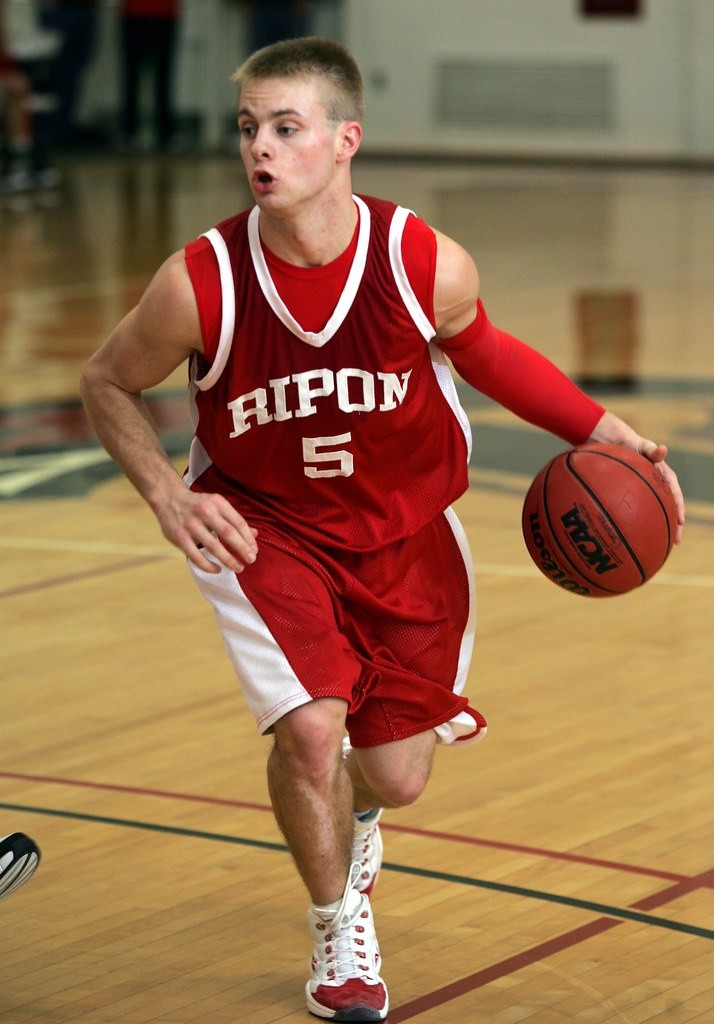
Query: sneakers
[305,862,389,1024]
[339,737,383,899]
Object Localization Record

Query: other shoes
[0,830,41,899]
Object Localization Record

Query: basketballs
[521,444,678,599]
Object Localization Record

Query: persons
[83,42,685,1021]
[0,0,187,194]
[225,0,343,54]
